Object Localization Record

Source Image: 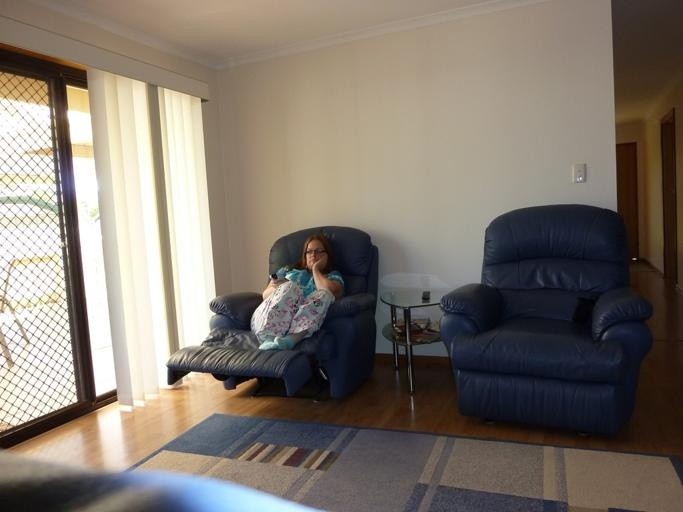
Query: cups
[421,286,431,300]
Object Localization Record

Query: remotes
[421,289,430,301]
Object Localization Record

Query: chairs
[438,203,654,439]
[165,226,379,399]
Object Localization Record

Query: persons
[249,233,345,351]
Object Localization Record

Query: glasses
[305,249,325,254]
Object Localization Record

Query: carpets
[122,412,683,512]
